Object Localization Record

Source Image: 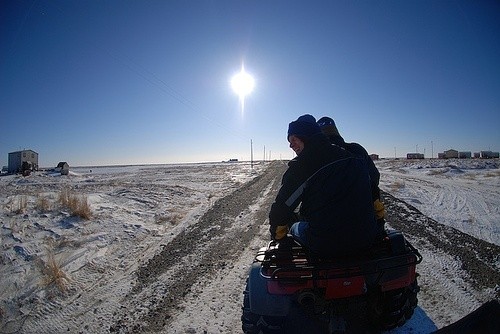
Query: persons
[317,117,380,188]
[266,114,381,256]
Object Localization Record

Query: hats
[316,116,339,136]
[287,114,321,137]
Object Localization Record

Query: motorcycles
[240,210,422,334]
